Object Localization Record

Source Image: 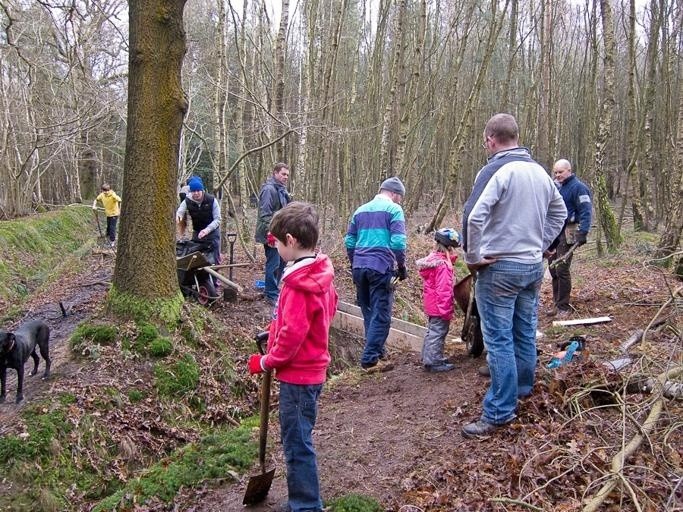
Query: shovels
[242,332,277,505]
[224,234,237,303]
[92,209,105,245]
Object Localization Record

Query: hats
[378,176,405,196]
[189,176,204,192]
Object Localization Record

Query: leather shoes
[546,307,557,315]
[478,365,489,377]
[483,380,492,387]
[379,353,388,361]
[553,308,572,319]
[430,362,454,373]
[442,357,448,363]
[460,420,506,439]
[360,360,394,375]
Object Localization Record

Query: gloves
[267,232,277,246]
[575,233,587,247]
[247,353,268,376]
[397,266,407,281]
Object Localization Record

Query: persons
[175,177,221,288]
[547,159,592,316]
[256,163,296,307]
[345,178,407,372]
[462,115,568,439]
[247,203,338,512]
[180,178,191,200]
[92,184,122,248]
[416,228,462,374]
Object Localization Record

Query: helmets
[434,226,463,248]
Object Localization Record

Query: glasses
[480,133,494,149]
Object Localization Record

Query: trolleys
[453,271,483,357]
[173,253,219,306]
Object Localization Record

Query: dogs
[0,320,51,404]
